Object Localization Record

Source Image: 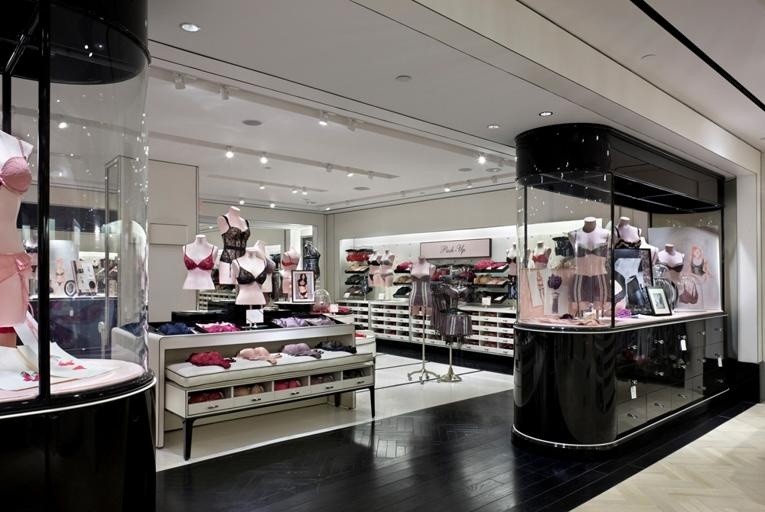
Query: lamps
[367,174,377,180]
[259,183,265,189]
[346,169,353,178]
[442,185,451,193]
[318,111,329,126]
[491,175,497,184]
[257,154,268,164]
[291,187,307,194]
[221,85,231,102]
[175,74,187,89]
[466,180,472,188]
[325,167,332,172]
[225,146,234,157]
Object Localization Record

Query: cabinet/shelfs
[0,0,155,512]
[110,269,377,464]
[511,123,731,462]
[337,249,515,372]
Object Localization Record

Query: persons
[181,204,325,309]
[531,214,716,314]
[0,128,39,328]
[369,249,383,287]
[380,249,396,288]
[407,257,438,316]
[506,242,531,275]
